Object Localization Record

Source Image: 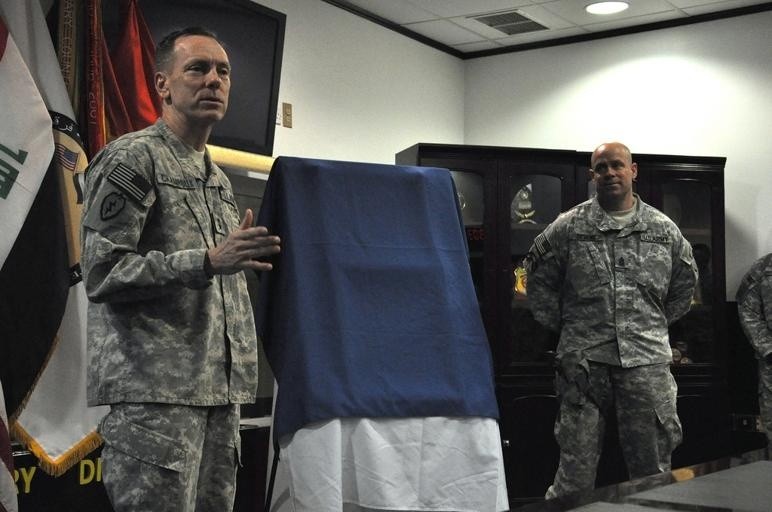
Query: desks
[501,447,772,512]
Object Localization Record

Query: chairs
[503,394,557,439]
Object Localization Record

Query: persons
[692,243,713,306]
[735,251,771,461]
[524,142,699,503]
[79,24,282,512]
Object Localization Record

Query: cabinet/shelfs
[395,143,578,439]
[577,151,727,397]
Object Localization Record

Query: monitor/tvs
[139,0,287,157]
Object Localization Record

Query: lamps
[583,0,632,20]
